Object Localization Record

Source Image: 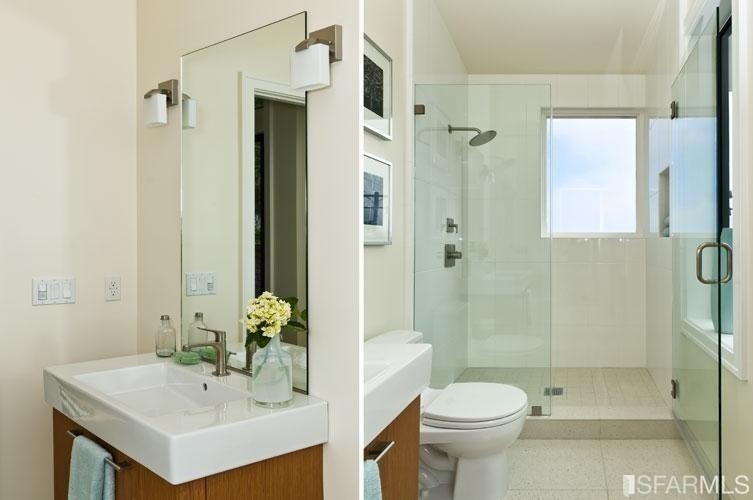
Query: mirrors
[178,11,309,396]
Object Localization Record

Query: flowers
[238,290,307,387]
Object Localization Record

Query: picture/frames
[362,32,394,247]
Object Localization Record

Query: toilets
[365,328,528,500]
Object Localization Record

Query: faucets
[241,339,258,371]
[182,326,232,377]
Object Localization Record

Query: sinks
[231,339,307,393]
[70,359,252,420]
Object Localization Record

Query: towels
[363,458,382,500]
[67,434,116,500]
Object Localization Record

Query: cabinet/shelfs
[363,393,418,500]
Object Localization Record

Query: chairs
[52,406,323,500]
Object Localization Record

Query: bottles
[189,312,208,348]
[156,315,176,357]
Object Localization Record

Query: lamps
[143,79,178,128]
[181,93,196,130]
[290,24,342,92]
